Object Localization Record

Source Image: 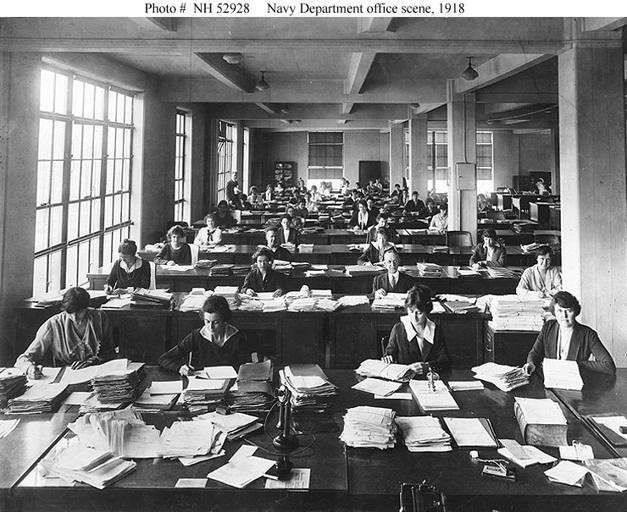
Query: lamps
[461,56,479,81]
[255,70,271,92]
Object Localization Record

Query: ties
[390,275,396,287]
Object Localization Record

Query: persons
[381,283,452,376]
[157,295,247,377]
[371,248,416,295]
[240,248,286,298]
[515,245,562,296]
[103,239,151,296]
[13,286,116,381]
[522,290,617,378]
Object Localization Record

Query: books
[511,398,572,448]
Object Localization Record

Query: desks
[0,186,626,511]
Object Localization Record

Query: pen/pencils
[188,352,193,365]
[27,359,45,377]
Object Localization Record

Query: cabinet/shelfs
[272,160,298,186]
